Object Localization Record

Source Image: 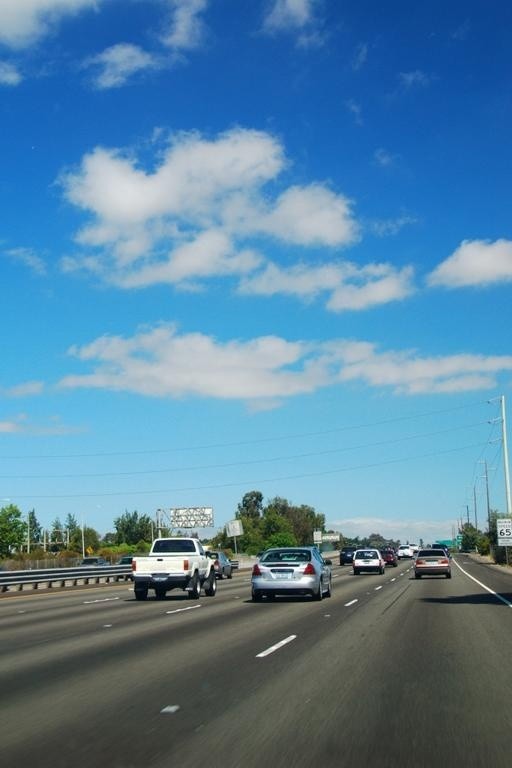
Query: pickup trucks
[131,537,217,600]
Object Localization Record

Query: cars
[78,538,234,579]
[340,543,452,578]
[251,547,332,600]
